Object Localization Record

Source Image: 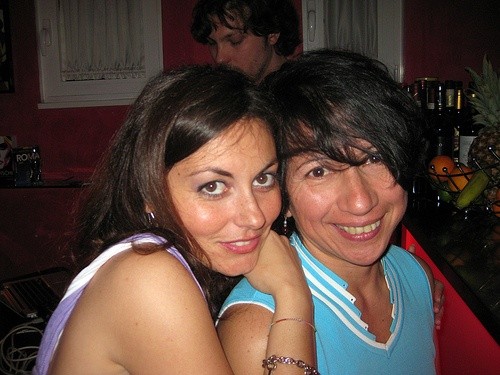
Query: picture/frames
[0,0,15,95]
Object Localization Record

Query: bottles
[29,149,42,184]
[393,76,482,165]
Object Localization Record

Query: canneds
[414,78,464,118]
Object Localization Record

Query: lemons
[438,181,453,203]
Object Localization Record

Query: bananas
[456,171,490,208]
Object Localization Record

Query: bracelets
[261,354,321,375]
[265,316,318,337]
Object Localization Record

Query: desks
[397,228,500,375]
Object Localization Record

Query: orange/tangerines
[429,155,473,191]
[486,188,500,218]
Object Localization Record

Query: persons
[214,48,436,375]
[189,0,303,87]
[32,62,446,375]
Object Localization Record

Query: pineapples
[463,53,500,186]
